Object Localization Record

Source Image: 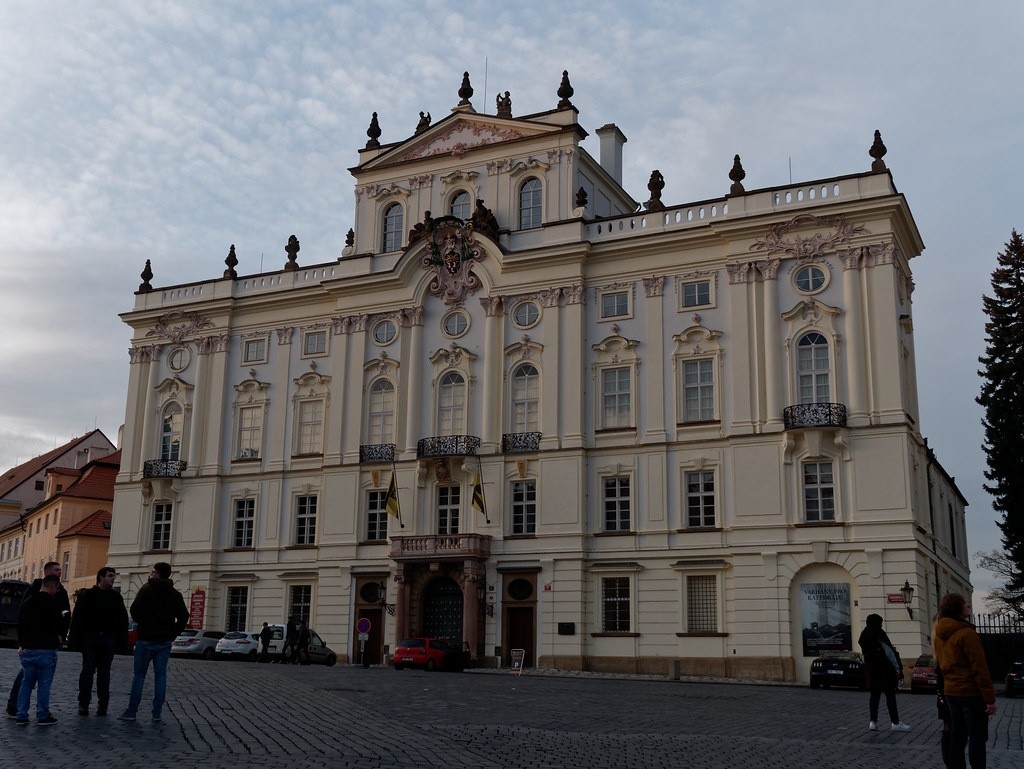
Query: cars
[170,629,227,660]
[810,650,869,692]
[1005,661,1024,700]
[908,653,939,694]
[214,631,260,662]
[393,636,472,673]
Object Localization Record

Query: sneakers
[892,721,912,732]
[78,710,89,716]
[37,716,58,725]
[869,721,881,731]
[97,711,107,717]
[16,719,30,725]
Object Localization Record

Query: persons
[5,560,71,720]
[931,593,996,769]
[116,562,190,721]
[858,614,912,731]
[279,616,299,665]
[16,574,64,726]
[70,567,129,717]
[287,623,312,666]
[256,622,274,664]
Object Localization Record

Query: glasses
[106,574,115,577]
[152,571,157,574]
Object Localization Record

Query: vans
[0,578,31,641]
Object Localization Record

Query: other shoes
[7,714,18,719]
[152,717,162,722]
[116,709,136,720]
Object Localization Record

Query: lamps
[899,579,915,620]
[376,578,396,617]
[485,602,495,618]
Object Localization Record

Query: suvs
[257,623,338,666]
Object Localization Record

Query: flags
[384,470,399,519]
[470,462,484,514]
